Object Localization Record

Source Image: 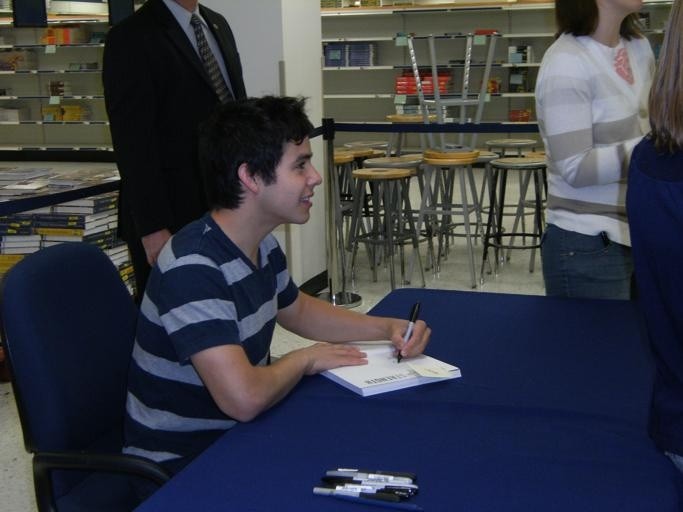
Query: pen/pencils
[397,301,420,363]
[311,465,424,511]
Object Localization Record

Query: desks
[131,288,680,512]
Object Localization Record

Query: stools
[327,121,551,291]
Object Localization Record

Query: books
[480,77,502,92]
[0,166,138,295]
[392,31,416,38]
[508,67,531,93]
[476,28,499,35]
[445,30,463,35]
[325,42,378,67]
[386,68,455,124]
[635,13,651,32]
[479,58,506,63]
[449,59,473,64]
[508,45,534,64]
[0,29,98,122]
[508,108,531,122]
[318,339,463,397]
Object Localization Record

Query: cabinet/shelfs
[0,19,110,162]
[321,1,674,125]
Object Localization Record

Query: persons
[119,94,434,503]
[534,0,657,300]
[103,0,247,307]
[624,0,682,477]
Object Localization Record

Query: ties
[188,13,235,108]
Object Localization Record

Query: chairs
[0,243,176,511]
